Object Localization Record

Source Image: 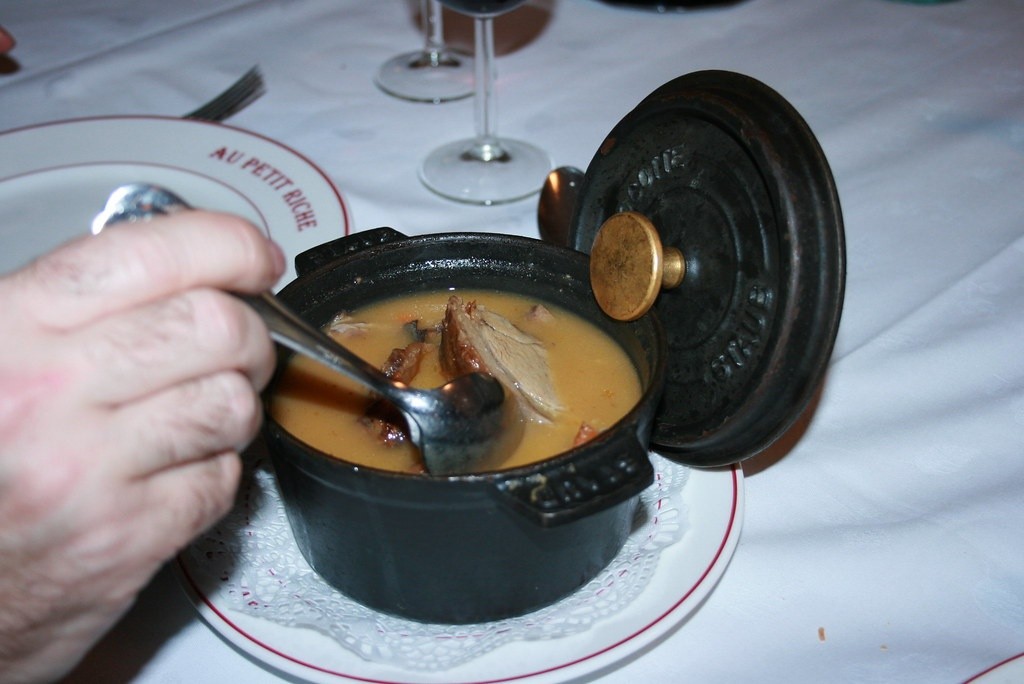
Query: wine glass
[376,0,487,102]
[420,0,553,204]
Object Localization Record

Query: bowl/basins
[263,226,663,626]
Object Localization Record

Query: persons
[0,25,288,684]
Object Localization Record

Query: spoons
[92,183,526,477]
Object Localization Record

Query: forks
[183,64,267,125]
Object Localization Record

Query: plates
[0,113,348,296]
[170,437,744,684]
